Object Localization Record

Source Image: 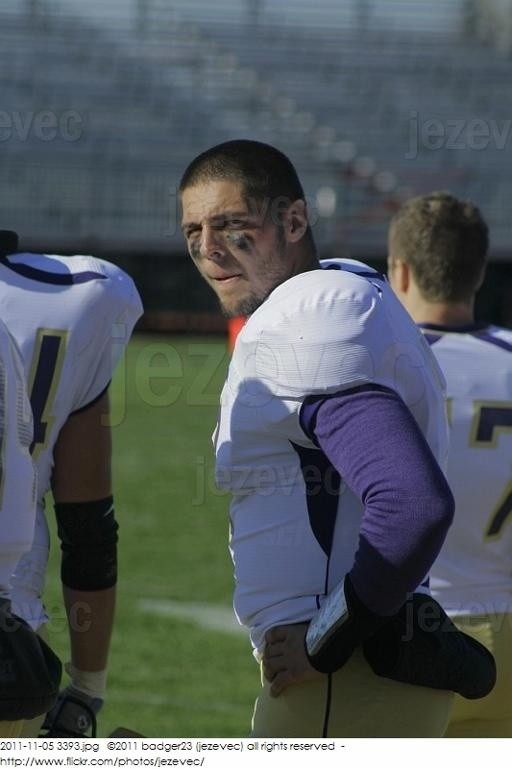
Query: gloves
[37,685,105,737]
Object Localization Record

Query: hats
[358,591,500,702]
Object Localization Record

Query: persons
[175,137,499,736]
[381,194,510,738]
[1,228,149,736]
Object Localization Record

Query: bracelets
[63,661,110,701]
